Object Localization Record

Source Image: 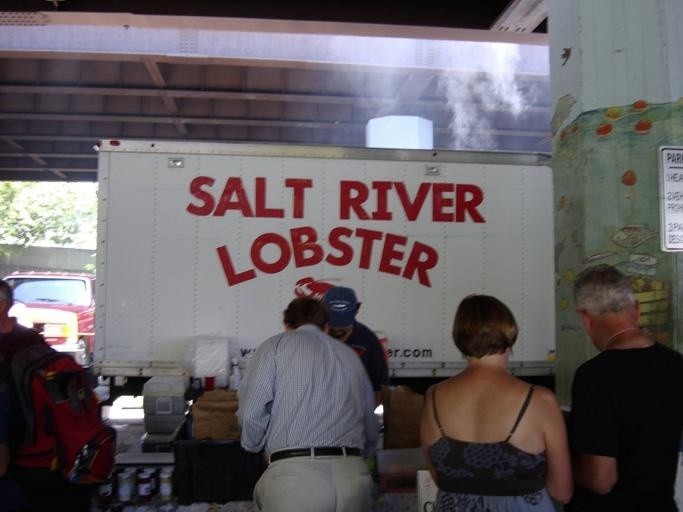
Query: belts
[270,445,362,464]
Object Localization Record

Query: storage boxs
[141,375,189,452]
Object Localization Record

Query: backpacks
[0,327,118,486]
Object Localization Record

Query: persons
[320,287,398,449]
[0,278,32,361]
[0,330,92,512]
[235,297,379,511]
[564,263,682,512]
[419,293,575,512]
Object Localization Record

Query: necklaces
[602,325,639,349]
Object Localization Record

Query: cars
[3,272,95,364]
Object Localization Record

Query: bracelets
[383,416,394,435]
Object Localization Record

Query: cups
[205,376,216,392]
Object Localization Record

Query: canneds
[97,467,175,504]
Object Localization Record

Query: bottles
[185,376,198,425]
[228,356,242,391]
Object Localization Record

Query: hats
[324,287,358,326]
